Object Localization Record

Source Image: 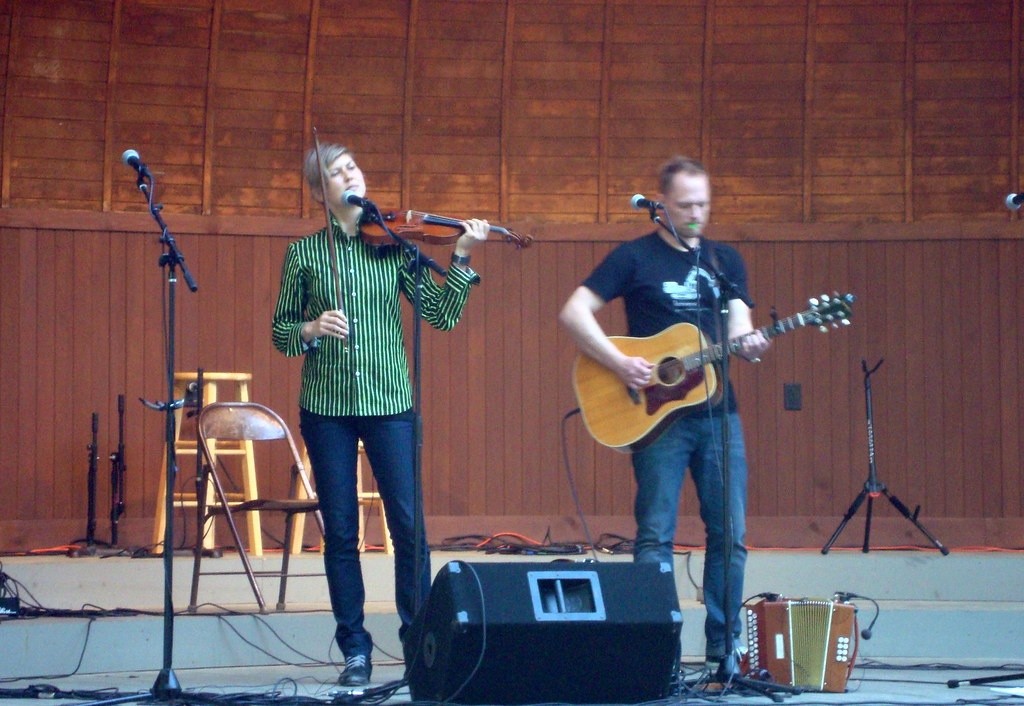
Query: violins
[357,207,533,248]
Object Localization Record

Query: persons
[558,156,772,677]
[273,142,490,687]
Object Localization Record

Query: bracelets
[452,252,471,266]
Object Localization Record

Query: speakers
[751,597,855,694]
[398,559,682,705]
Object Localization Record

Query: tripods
[89,176,245,706]
[821,359,950,557]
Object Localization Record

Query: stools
[152,371,266,557]
[289,437,394,556]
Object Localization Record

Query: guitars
[570,292,856,455]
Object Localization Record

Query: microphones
[340,191,373,208]
[120,149,152,177]
[632,193,664,212]
[838,591,880,639]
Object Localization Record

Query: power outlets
[784,382,801,410]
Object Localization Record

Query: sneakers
[338,655,372,686]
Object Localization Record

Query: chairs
[188,401,325,614]
